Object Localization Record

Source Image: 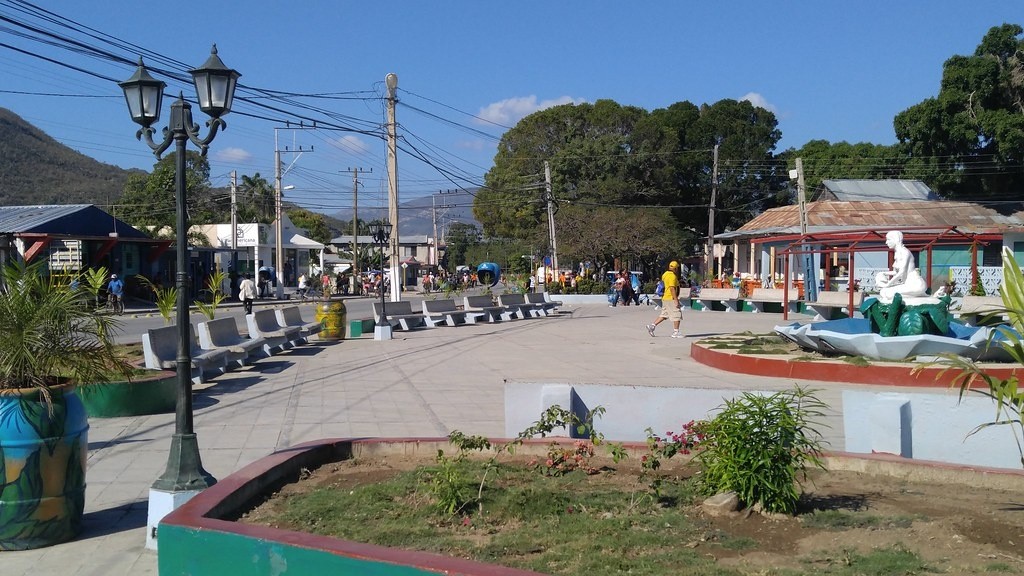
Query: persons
[547,271,582,287]
[608,268,641,308]
[875,230,930,299]
[285,260,292,287]
[422,270,477,293]
[259,274,266,300]
[721,270,726,288]
[239,272,255,315]
[297,270,381,298]
[106,274,123,313]
[646,261,685,338]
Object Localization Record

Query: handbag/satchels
[257,282,262,287]
[239,284,245,301]
[655,281,665,297]
[633,286,641,294]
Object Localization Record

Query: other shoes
[609,305,616,308]
[303,294,308,298]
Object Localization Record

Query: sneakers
[671,330,686,339]
[646,324,655,337]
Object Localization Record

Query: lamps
[693,243,714,254]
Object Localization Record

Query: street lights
[117,41,244,552]
[383,71,401,302]
[275,185,295,299]
[367,218,394,339]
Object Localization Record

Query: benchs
[198,317,268,370]
[141,323,232,385]
[372,301,427,332]
[949,295,1009,324]
[525,292,563,318]
[690,288,741,311]
[422,300,470,327]
[745,289,800,314]
[804,291,870,322]
[463,295,507,324]
[499,293,536,321]
[246,307,300,356]
[276,306,323,347]
[650,288,691,309]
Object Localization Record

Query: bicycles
[299,288,306,304]
[105,289,125,317]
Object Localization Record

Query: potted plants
[1,254,135,552]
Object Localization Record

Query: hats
[463,273,467,275]
[548,273,552,276]
[669,261,680,270]
